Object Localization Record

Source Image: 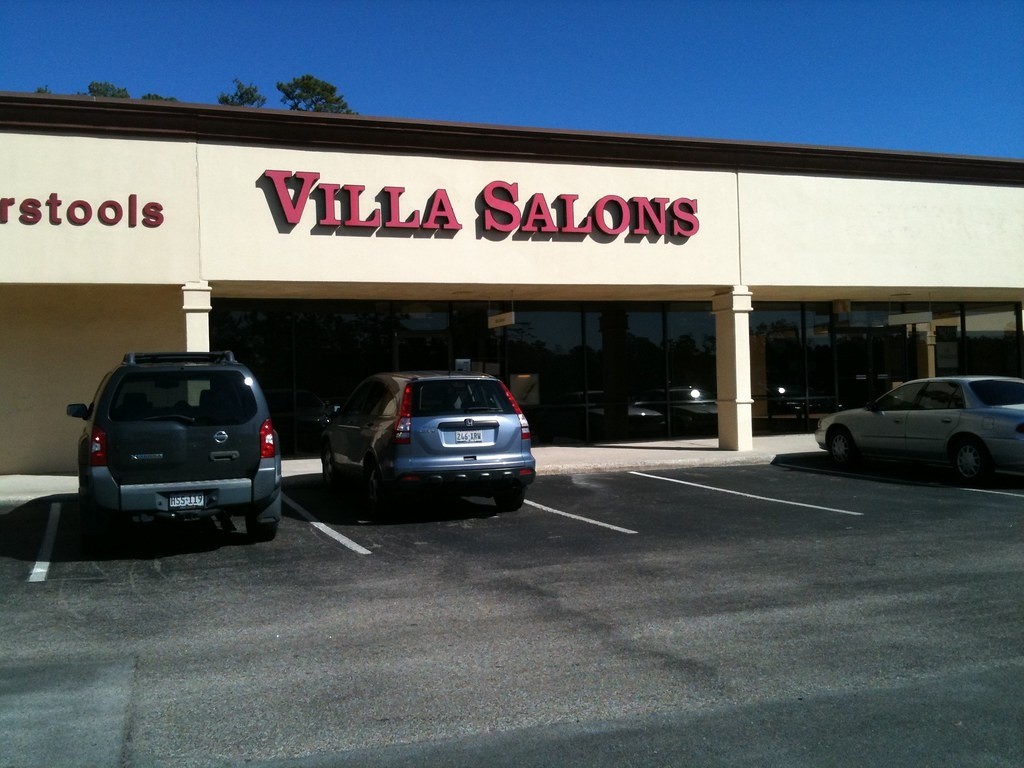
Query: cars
[556,391,666,437]
[320,372,537,512]
[815,376,1024,475]
[265,390,326,451]
[665,388,718,436]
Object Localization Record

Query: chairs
[200,389,229,415]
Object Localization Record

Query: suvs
[67,352,281,540]
